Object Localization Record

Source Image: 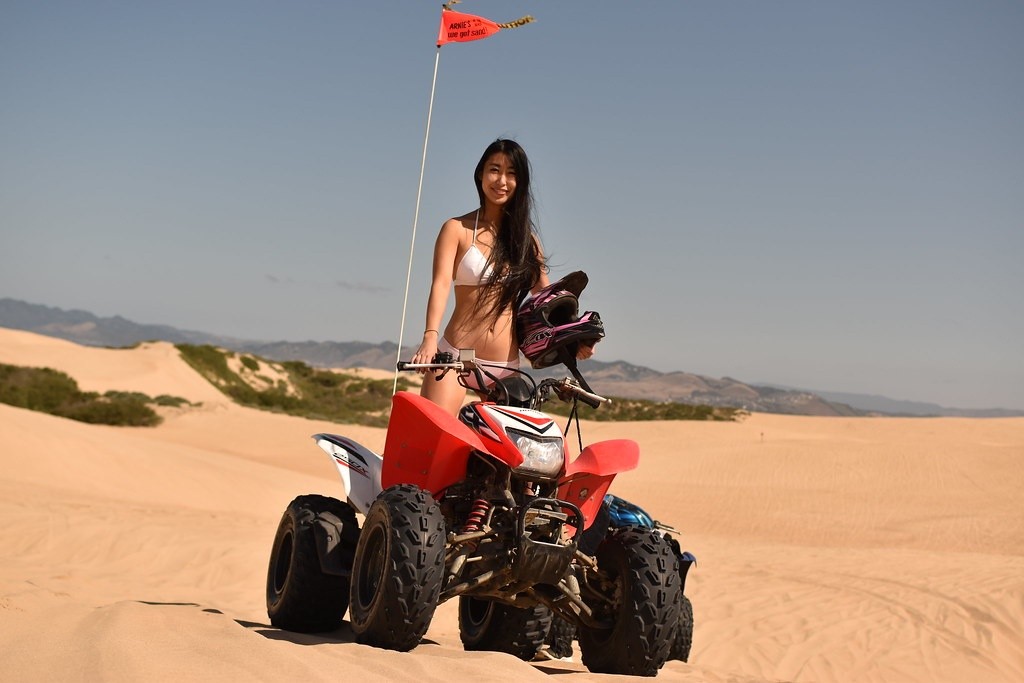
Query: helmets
[517,270,606,370]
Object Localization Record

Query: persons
[411,139,596,419]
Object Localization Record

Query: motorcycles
[266,344,697,678]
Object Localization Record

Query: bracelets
[422,329,440,335]
